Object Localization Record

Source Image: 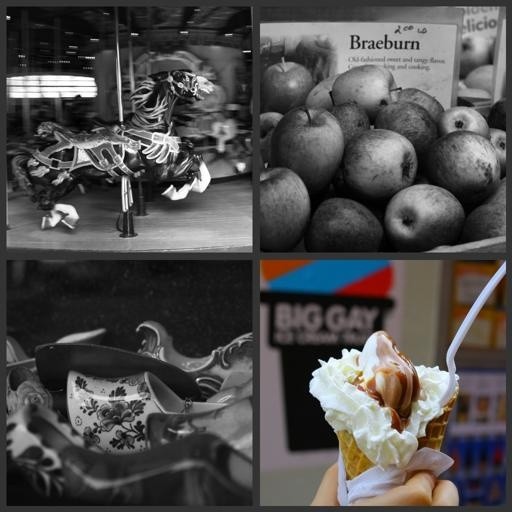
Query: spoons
[430,260,507,408]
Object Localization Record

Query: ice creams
[308,329,461,481]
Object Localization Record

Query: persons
[10,65,218,231]
[304,463,460,506]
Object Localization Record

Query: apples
[260,56,506,252]
[458,33,494,100]
